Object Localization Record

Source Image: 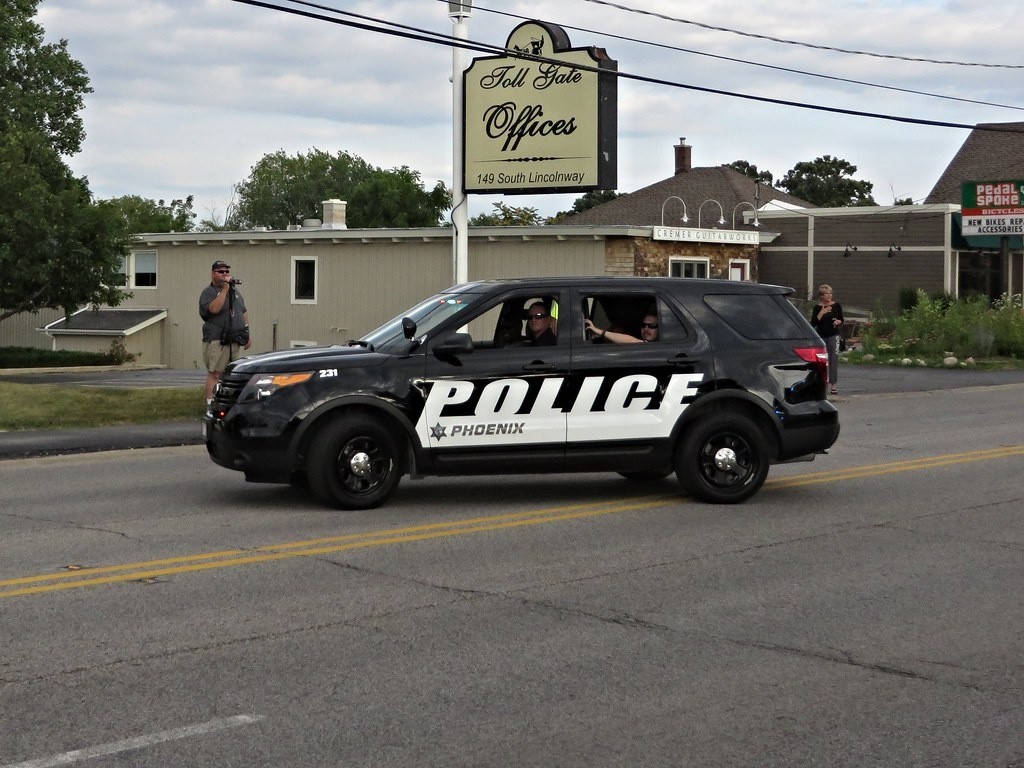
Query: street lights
[449,0,472,334]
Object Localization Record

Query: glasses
[640,322,658,329]
[213,270,229,274]
[528,313,549,319]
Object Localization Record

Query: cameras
[221,276,242,287]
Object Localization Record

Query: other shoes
[831,389,837,394]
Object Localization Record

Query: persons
[810,284,844,394]
[585,314,659,344]
[198,261,251,415]
[528,300,557,346]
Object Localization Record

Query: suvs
[200,274,839,510]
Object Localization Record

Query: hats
[212,261,231,270]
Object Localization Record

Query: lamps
[843,243,857,257]
[699,199,727,228]
[733,202,760,230]
[661,196,690,226]
[887,243,901,258]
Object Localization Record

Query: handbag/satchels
[840,338,845,352]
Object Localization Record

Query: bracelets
[600,330,607,336]
